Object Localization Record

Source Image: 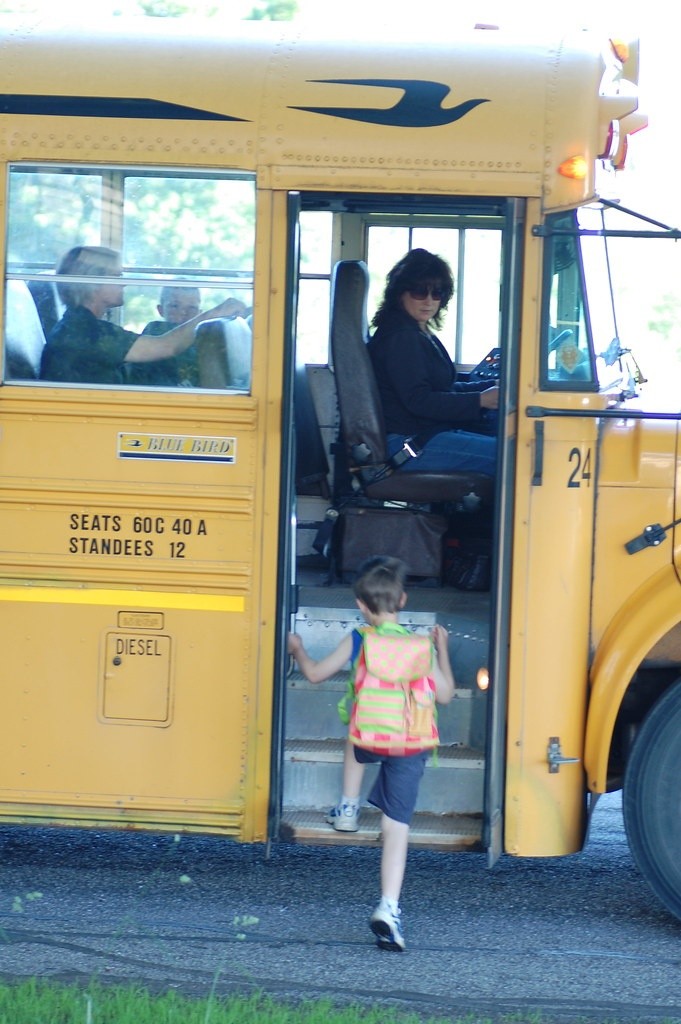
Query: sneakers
[326,797,360,831]
[368,905,406,952]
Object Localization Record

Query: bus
[0,16,681,919]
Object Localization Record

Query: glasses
[403,283,444,300]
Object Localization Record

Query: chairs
[318,257,469,589]
[4,279,46,383]
[21,271,68,341]
[191,317,252,392]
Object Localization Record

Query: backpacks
[337,625,440,768]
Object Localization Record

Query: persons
[38,246,247,382]
[288,554,456,951]
[367,248,500,477]
[128,277,203,389]
